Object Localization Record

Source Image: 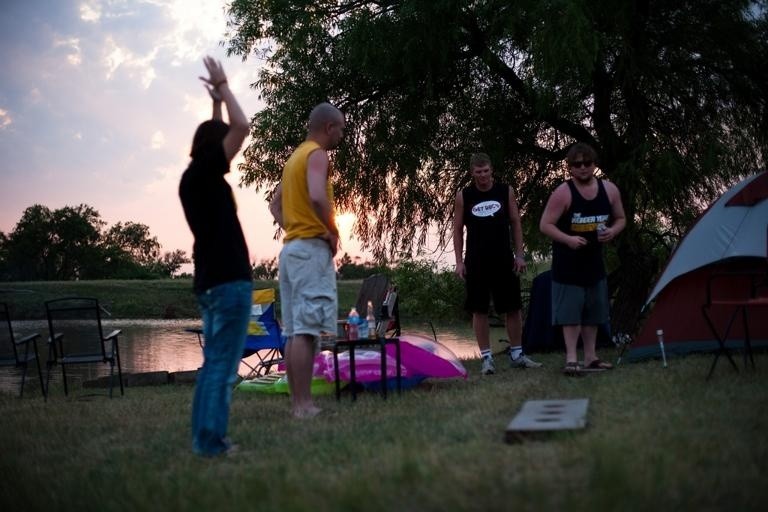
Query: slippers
[564,362,581,374]
[585,359,613,369]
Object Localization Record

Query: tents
[625,171,768,364]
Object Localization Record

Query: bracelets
[216,80,227,90]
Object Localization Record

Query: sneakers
[481,355,496,375]
[508,352,544,368]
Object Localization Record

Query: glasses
[573,160,594,168]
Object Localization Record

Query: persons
[454,152,543,375]
[539,142,627,377]
[178,56,255,459]
[270,102,347,421]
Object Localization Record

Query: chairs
[45,296,124,400]
[339,273,401,337]
[0,301,46,399]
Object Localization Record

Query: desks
[185,326,287,393]
[701,271,768,381]
[321,337,401,402]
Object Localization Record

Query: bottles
[349,307,359,338]
[366,300,377,339]
[597,223,606,240]
